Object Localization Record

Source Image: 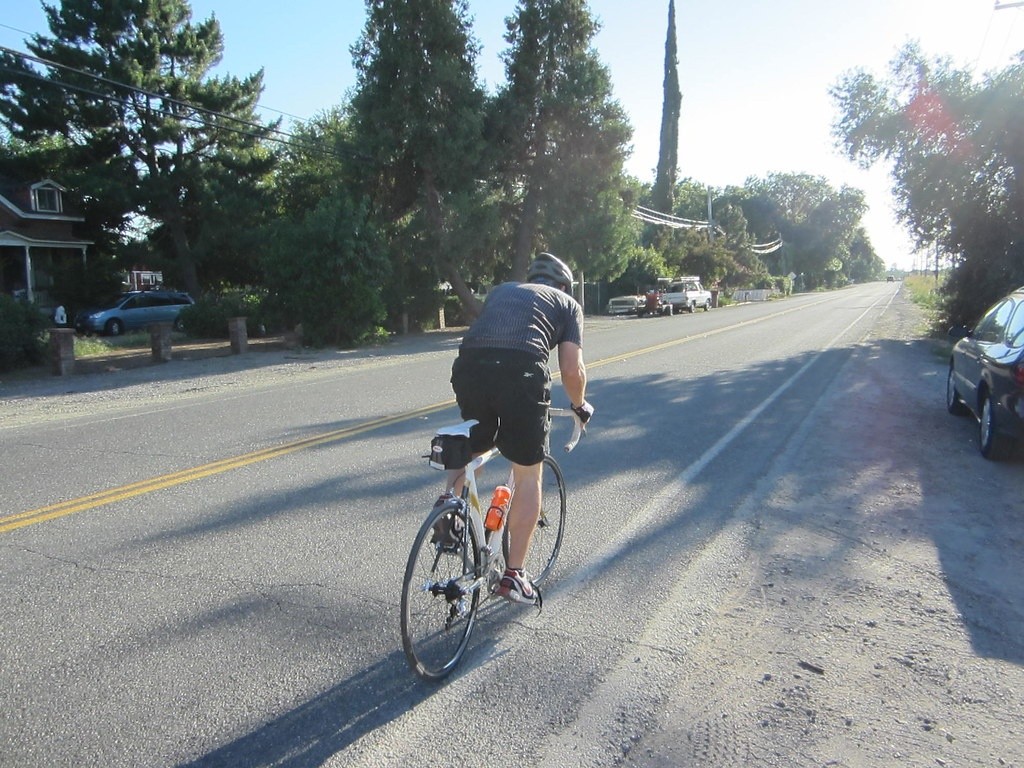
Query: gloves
[572,399,595,424]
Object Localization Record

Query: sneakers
[496,570,537,605]
[432,495,465,547]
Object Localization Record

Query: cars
[887,276,894,281]
[606,289,673,317]
[73,289,195,336]
[946,283,1023,457]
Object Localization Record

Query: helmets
[526,251,574,293]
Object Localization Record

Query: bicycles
[399,404,581,681]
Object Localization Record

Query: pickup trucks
[659,280,712,313]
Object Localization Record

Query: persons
[428,251,592,610]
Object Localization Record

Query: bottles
[484,483,511,531]
